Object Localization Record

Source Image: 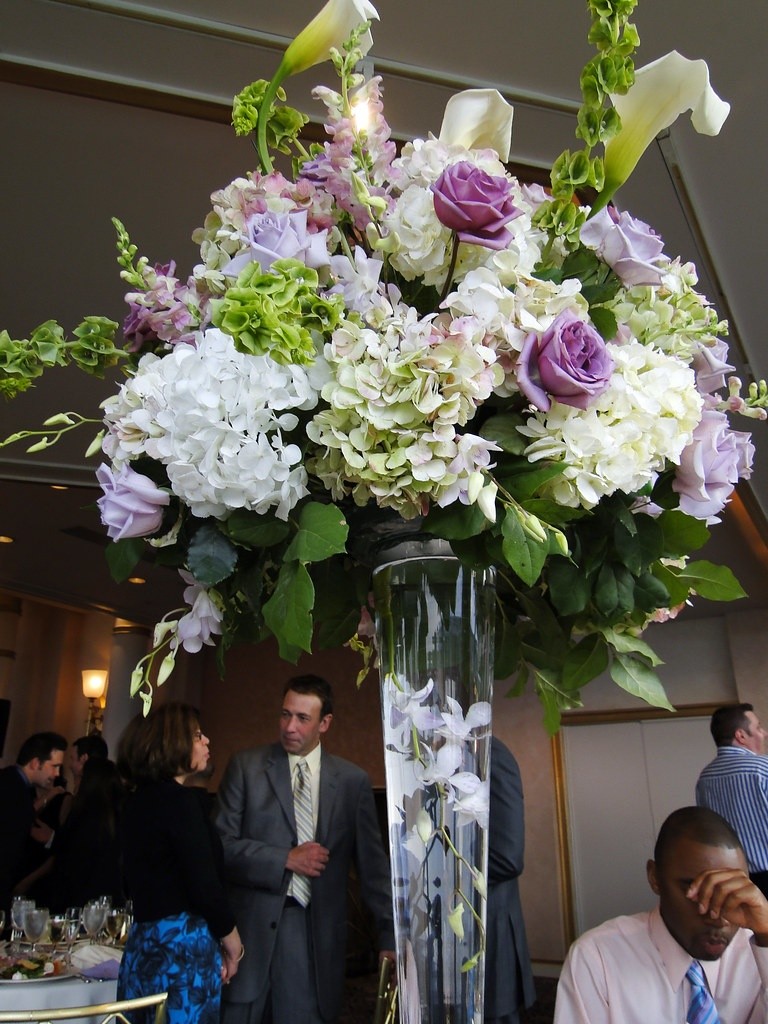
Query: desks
[0,940,128,1024]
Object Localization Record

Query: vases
[368,527,501,1024]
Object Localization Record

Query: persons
[0,730,116,923]
[114,701,245,1024]
[211,674,394,1024]
[695,703,768,899]
[484,736,537,1024]
[554,807,768,1024]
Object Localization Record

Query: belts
[284,896,300,907]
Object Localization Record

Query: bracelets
[225,944,245,963]
[43,795,47,806]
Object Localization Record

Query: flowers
[0,0,768,728]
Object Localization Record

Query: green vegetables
[0,956,46,981]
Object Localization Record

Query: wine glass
[11,896,134,970]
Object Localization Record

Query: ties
[685,960,720,1024]
[292,759,314,909]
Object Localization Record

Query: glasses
[192,729,202,741]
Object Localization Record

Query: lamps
[80,669,108,730]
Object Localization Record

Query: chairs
[0,992,170,1024]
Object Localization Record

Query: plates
[0,969,74,984]
[13,938,90,945]
